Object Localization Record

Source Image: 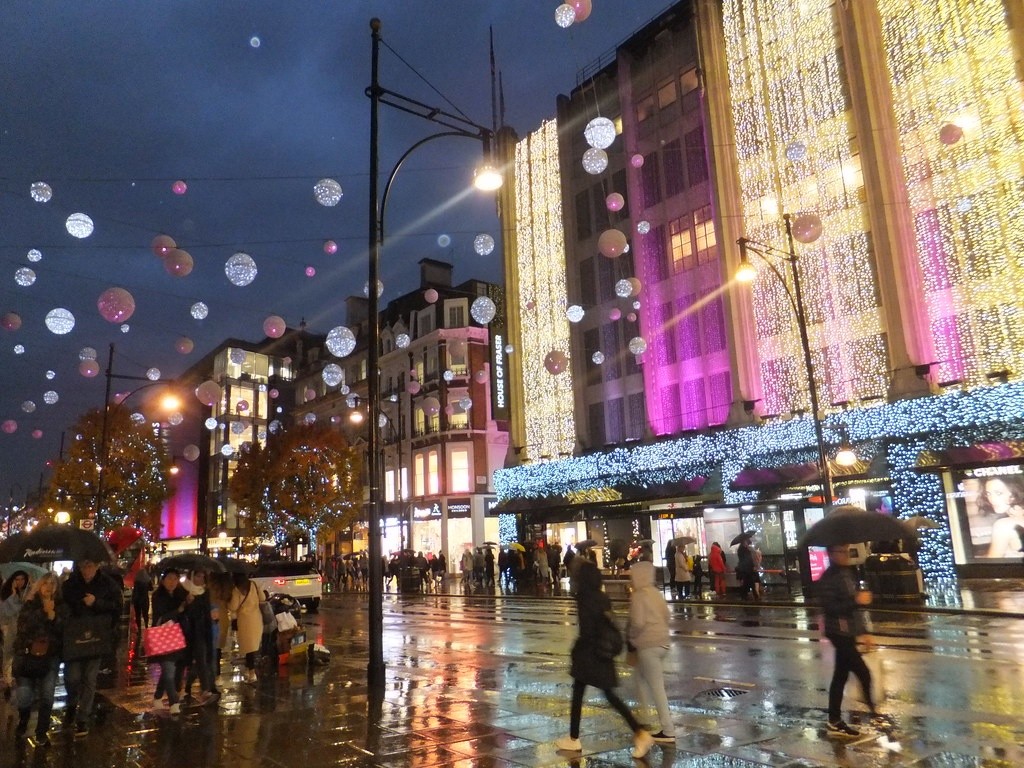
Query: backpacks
[591,614,623,659]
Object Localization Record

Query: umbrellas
[799,500,942,558]
[637,539,656,548]
[472,541,542,553]
[153,552,230,585]
[670,536,696,548]
[731,530,757,547]
[0,523,113,577]
[575,539,598,551]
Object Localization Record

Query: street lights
[93,343,187,531]
[737,213,837,516]
[347,392,410,560]
[358,14,503,699]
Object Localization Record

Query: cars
[249,560,327,611]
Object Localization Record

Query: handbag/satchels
[259,602,274,625]
[276,611,297,631]
[14,654,49,679]
[144,620,187,656]
[63,613,112,662]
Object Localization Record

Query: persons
[149,571,269,715]
[666,539,764,603]
[817,543,878,738]
[295,546,565,593]
[0,561,122,747]
[131,568,154,633]
[258,597,280,677]
[974,474,1024,557]
[555,555,656,758]
[623,560,677,744]
[563,538,601,578]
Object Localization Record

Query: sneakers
[827,721,861,738]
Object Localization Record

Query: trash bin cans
[848,543,867,591]
[866,554,924,605]
[399,567,422,594]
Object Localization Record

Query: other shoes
[169,703,180,714]
[76,722,90,736]
[36,733,51,745]
[245,674,257,683]
[632,733,654,758]
[554,734,581,750]
[152,700,163,709]
[652,730,675,742]
[64,706,76,724]
[15,736,28,751]
[190,692,220,708]
